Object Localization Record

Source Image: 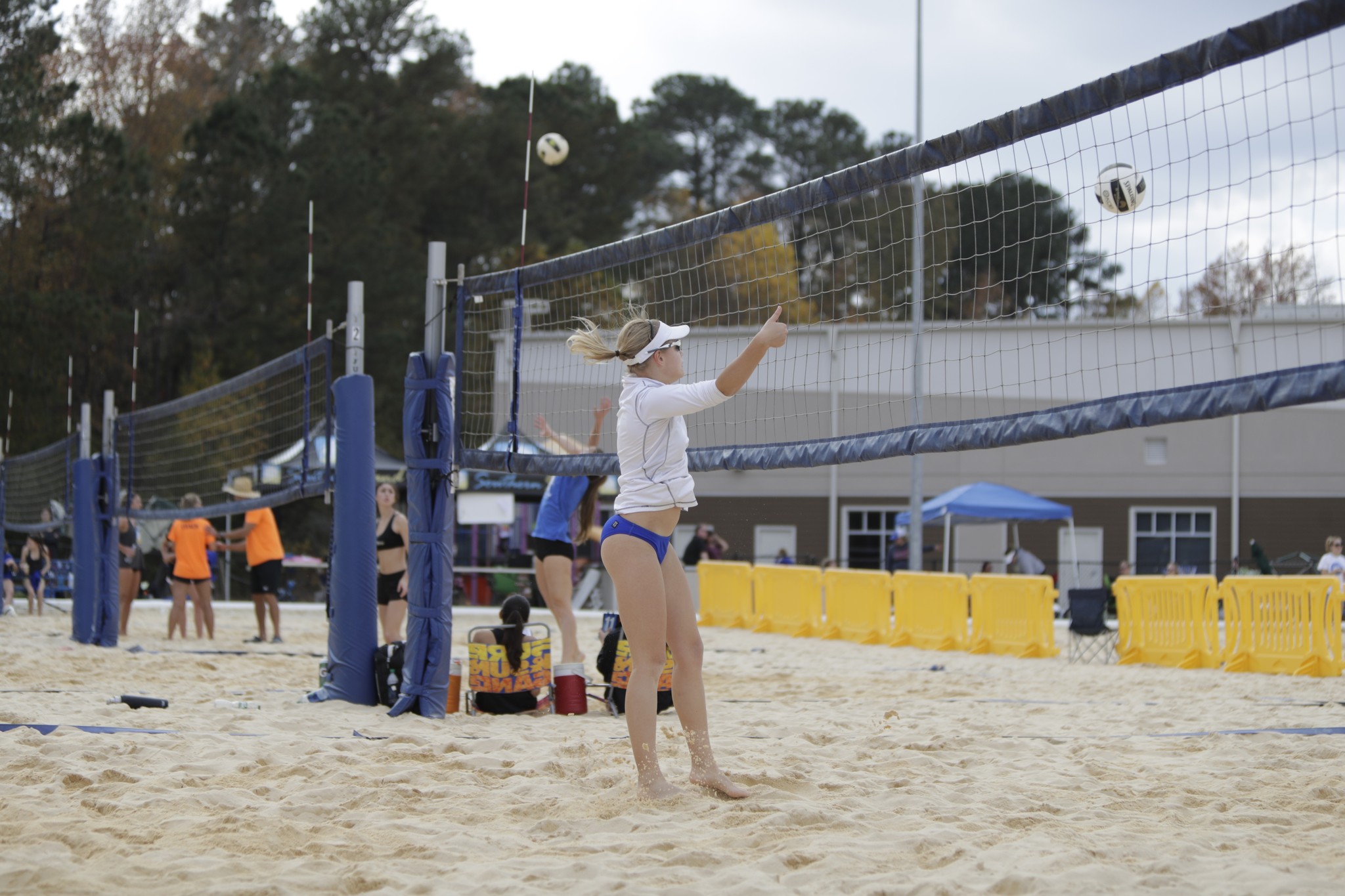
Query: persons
[983,534,1345,621]
[3,476,729,715]
[566,305,788,802]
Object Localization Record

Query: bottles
[319,657,328,688]
[388,670,398,706]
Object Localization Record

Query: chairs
[1066,587,1119,667]
[464,621,555,717]
[609,627,676,719]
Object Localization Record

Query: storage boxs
[446,660,462,715]
[552,662,588,716]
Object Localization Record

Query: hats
[221,476,261,499]
[623,320,690,366]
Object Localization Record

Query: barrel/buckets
[446,660,462,712]
[553,663,594,714]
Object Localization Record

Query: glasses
[1330,543,1344,547]
[645,340,681,354]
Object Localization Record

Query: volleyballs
[536,133,569,166]
[1094,163,1145,213]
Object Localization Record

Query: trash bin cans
[1069,587,1108,632]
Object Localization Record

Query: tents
[222,417,404,601]
[457,423,622,499]
[895,481,1083,590]
